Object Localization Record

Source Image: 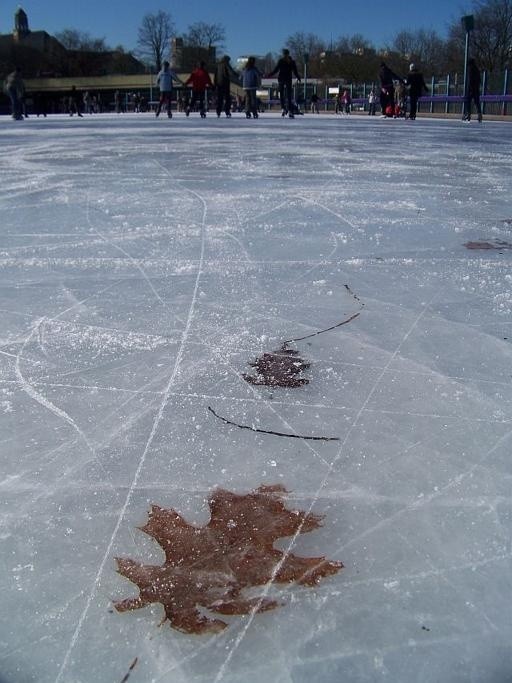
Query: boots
[156,109,258,119]
[477,113,482,121]
[281,112,294,118]
[379,114,416,119]
[462,113,471,121]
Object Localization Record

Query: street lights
[459,13,476,116]
[301,51,310,99]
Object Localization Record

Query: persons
[367,90,375,115]
[6,65,47,120]
[115,88,144,113]
[68,85,97,117]
[310,95,324,114]
[335,87,351,114]
[156,49,304,118]
[377,62,429,120]
[462,58,482,122]
